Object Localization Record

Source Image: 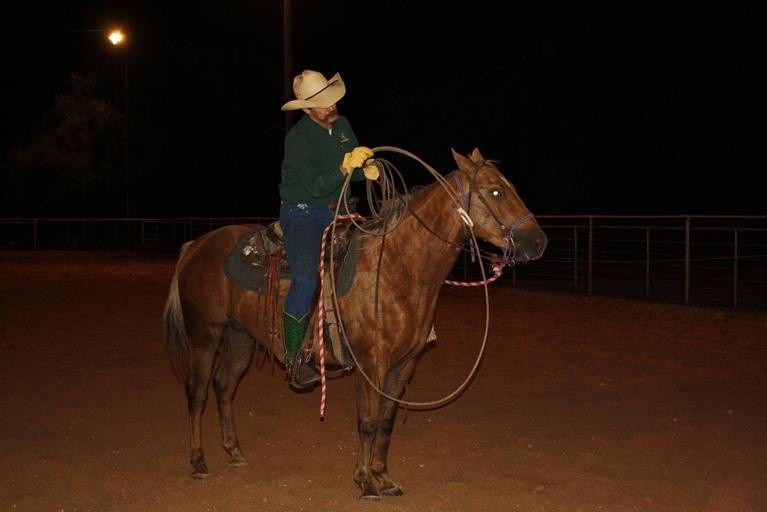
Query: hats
[281,70,346,111]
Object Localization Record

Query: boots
[283,310,321,386]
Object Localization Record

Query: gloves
[342,146,380,181]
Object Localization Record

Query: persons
[278,69,379,388]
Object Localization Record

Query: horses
[161,142,550,502]
